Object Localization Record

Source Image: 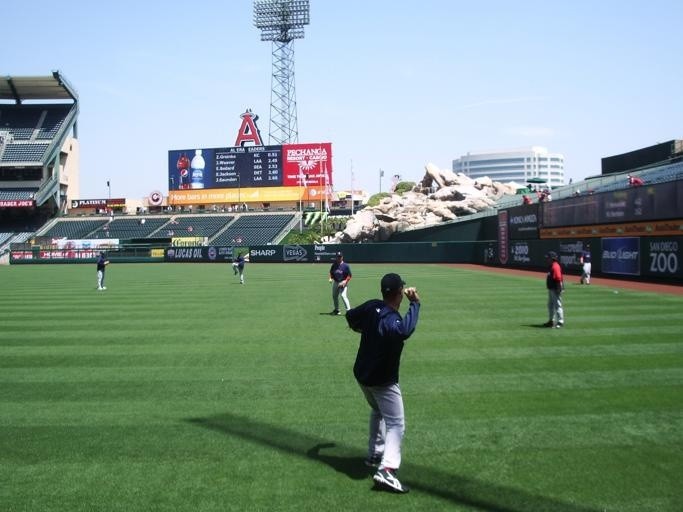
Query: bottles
[177,150,205,190]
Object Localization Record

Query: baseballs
[329,279,333,283]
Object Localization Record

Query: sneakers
[329,309,342,315]
[233,270,239,276]
[579,278,590,285]
[240,279,246,286]
[543,320,564,328]
[363,456,381,467]
[372,464,409,494]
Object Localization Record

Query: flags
[299,170,306,187]
[380,170,383,177]
[324,168,330,185]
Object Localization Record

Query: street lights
[235,173,241,214]
[304,173,310,209]
[106,180,111,198]
[169,177,174,212]
[378,169,383,193]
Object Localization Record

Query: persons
[344,271,422,495]
[521,185,555,205]
[326,251,352,315]
[625,174,644,186]
[95,202,247,243]
[230,249,244,285]
[94,251,110,292]
[579,243,593,285]
[540,250,566,330]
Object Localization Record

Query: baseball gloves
[338,280,348,288]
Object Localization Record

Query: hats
[336,251,343,257]
[544,251,558,260]
[381,272,406,294]
